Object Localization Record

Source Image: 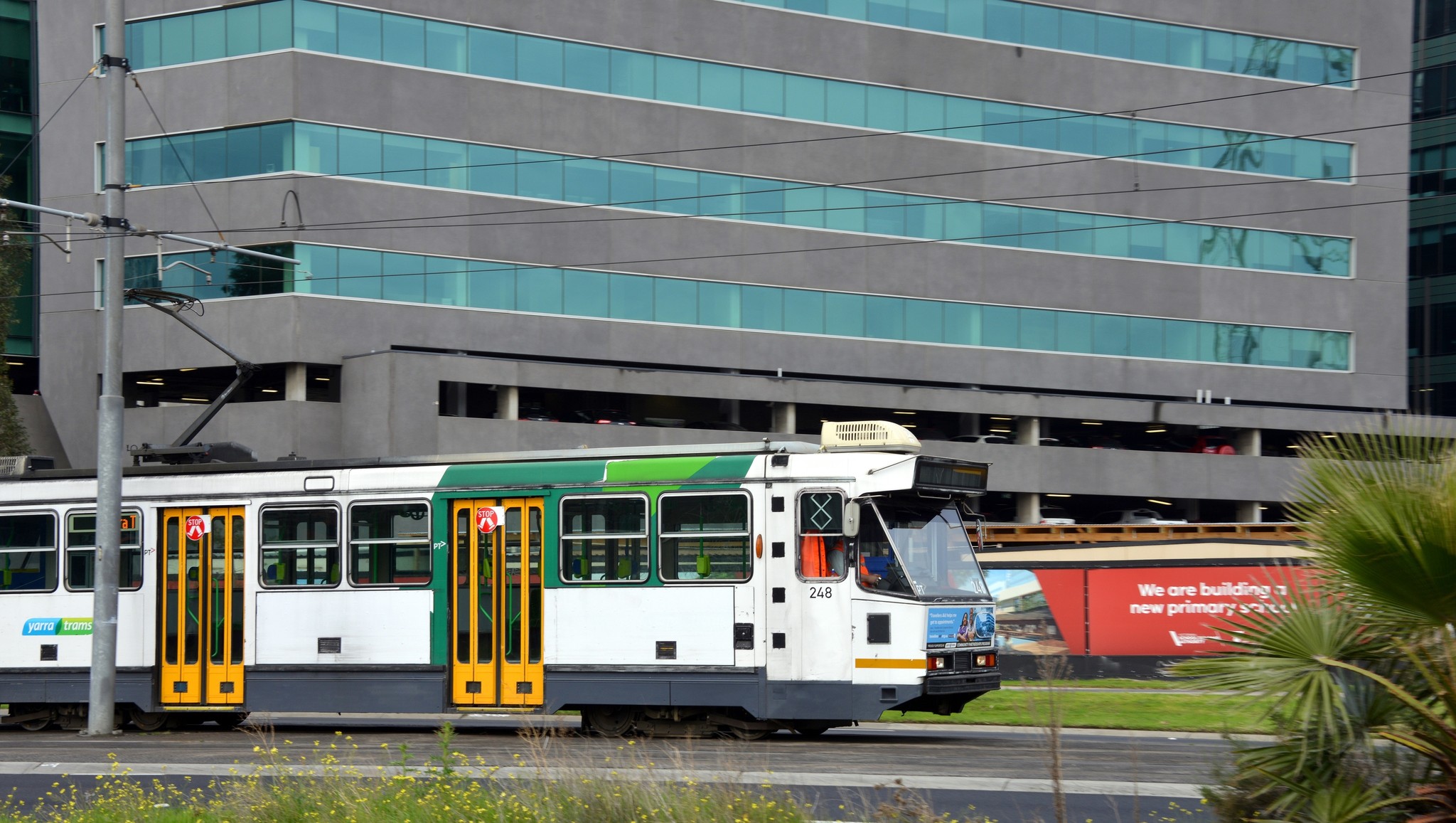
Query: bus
[0,418,1005,738]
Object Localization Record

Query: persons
[957,608,976,641]
[825,529,882,588]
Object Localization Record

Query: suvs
[1013,507,1075,535]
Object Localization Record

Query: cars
[1040,437,1062,446]
[492,407,560,422]
[1114,507,1163,533]
[954,435,1006,444]
[570,412,636,425]
[1196,443,1236,454]
